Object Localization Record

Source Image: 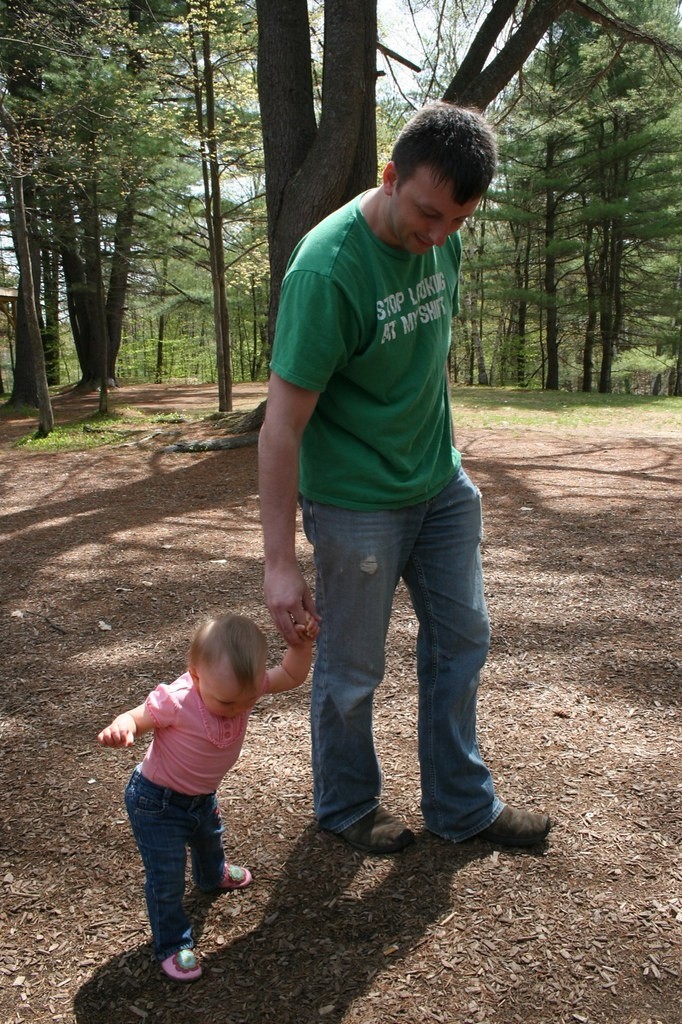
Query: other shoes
[471,806,551,846]
[341,805,412,854]
[160,949,203,983]
[218,863,252,889]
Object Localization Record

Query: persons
[97,611,321,982]
[257,107,553,853]
